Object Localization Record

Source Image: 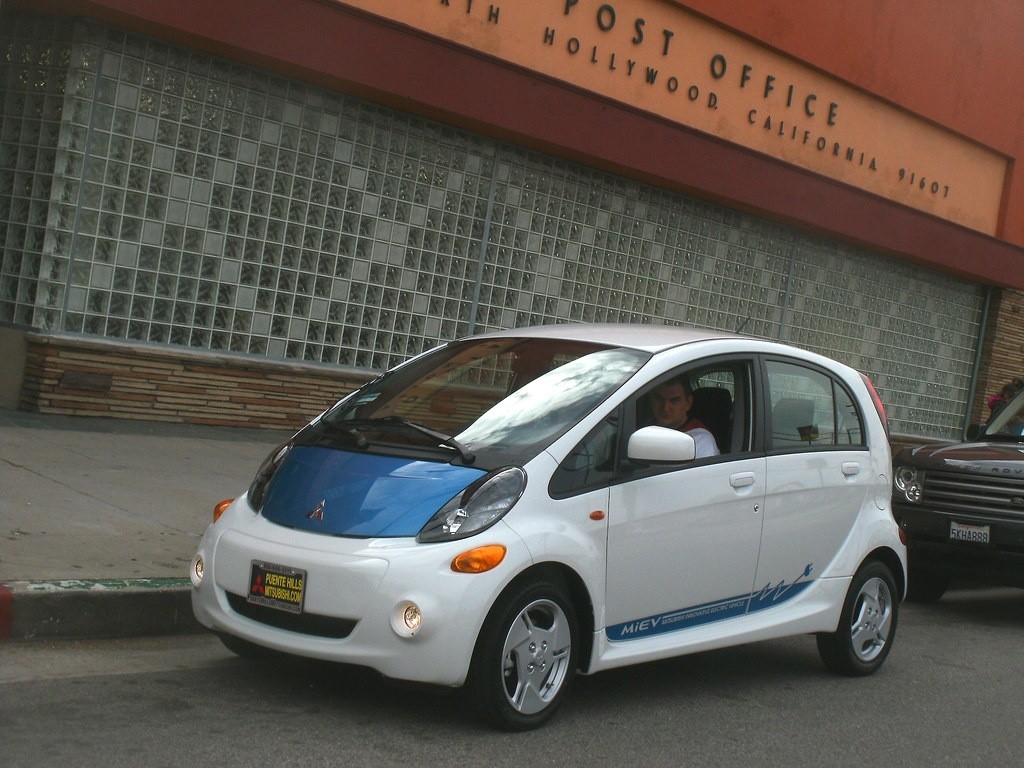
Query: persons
[987,379,1024,423]
[646,374,720,459]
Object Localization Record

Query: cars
[188,322,907,729]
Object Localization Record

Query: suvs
[894,376,1021,602]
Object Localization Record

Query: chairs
[770,399,813,450]
[687,384,733,456]
